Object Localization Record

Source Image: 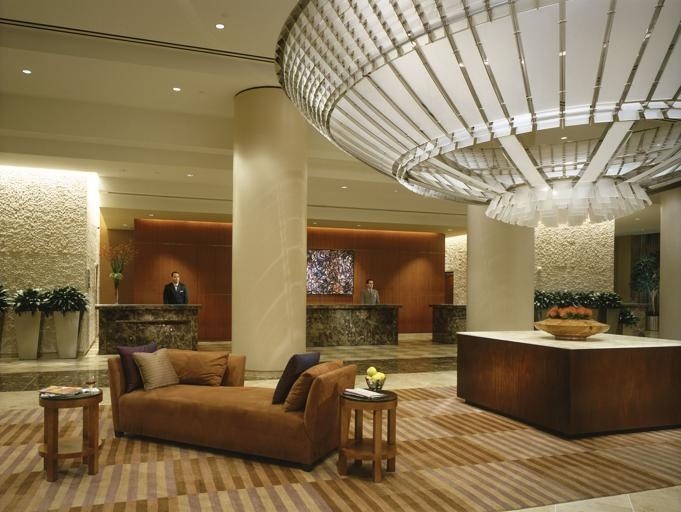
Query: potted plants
[0,286,10,341]
[534,291,550,321]
[599,292,624,334]
[36,285,90,359]
[7,288,49,360]
[618,310,640,336]
[581,291,599,321]
[629,256,659,338]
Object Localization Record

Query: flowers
[103,239,135,299]
[546,306,593,319]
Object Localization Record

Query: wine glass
[85,375,97,393]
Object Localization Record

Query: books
[343,387,387,399]
[38,385,83,400]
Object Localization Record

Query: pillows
[283,359,343,412]
[272,352,320,404]
[116,343,230,393]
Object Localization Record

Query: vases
[533,319,609,341]
[113,279,118,304]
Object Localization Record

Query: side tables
[38,387,103,482]
[339,389,398,482]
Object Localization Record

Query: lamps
[275,0,680,228]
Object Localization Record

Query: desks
[455,330,681,440]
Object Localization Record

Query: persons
[360,279,380,304]
[163,272,188,304]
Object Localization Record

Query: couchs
[108,355,357,472]
